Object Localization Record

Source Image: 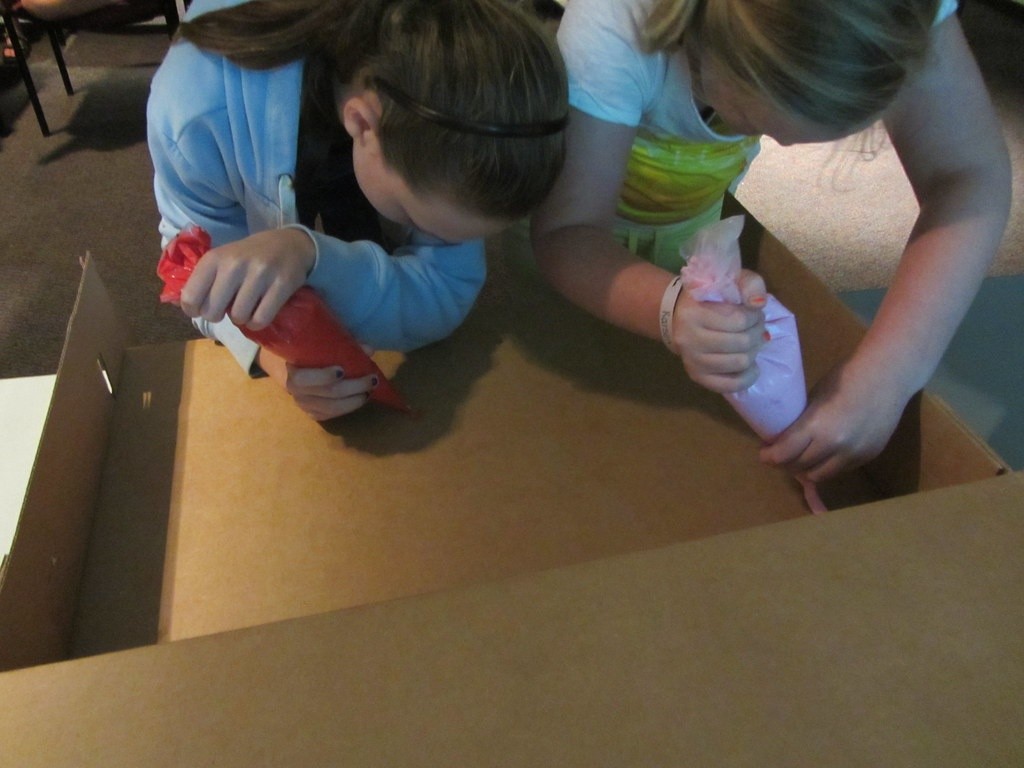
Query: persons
[533,0,1015,490]
[147,1,574,423]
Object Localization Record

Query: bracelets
[660,276,688,357]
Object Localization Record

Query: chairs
[0,0,189,139]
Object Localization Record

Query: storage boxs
[6,191,1024,766]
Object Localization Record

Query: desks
[0,358,119,564]
